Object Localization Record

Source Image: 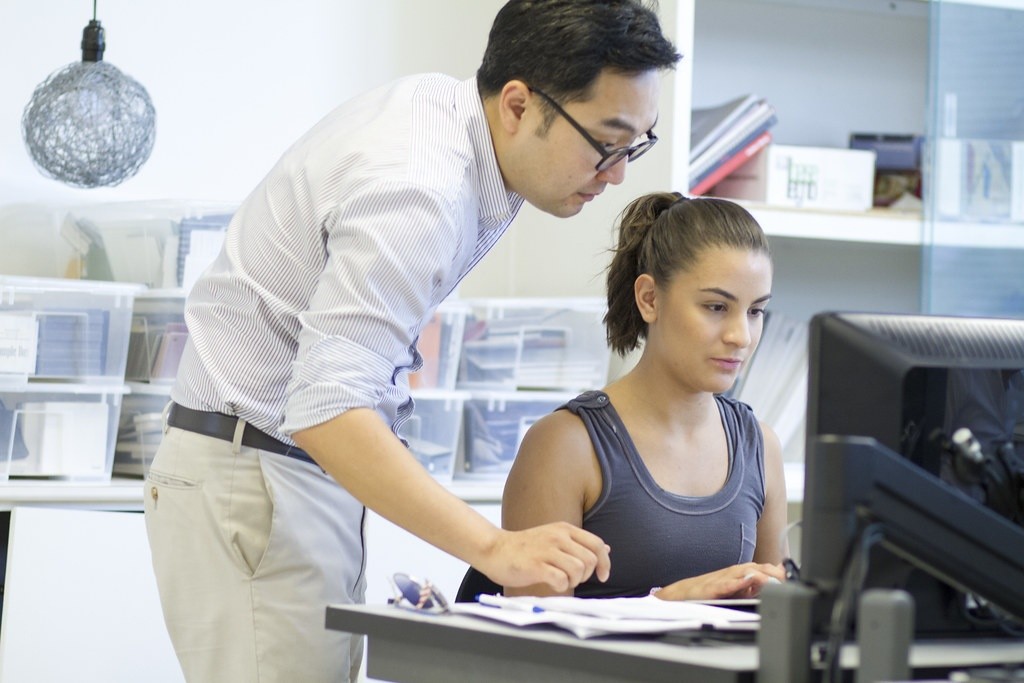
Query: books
[460,314,567,385]
[112,407,169,476]
[124,321,187,380]
[688,94,778,197]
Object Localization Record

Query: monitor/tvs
[805,310,1023,644]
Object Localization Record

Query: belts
[168,403,317,465]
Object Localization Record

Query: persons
[501,192,790,601]
[144,1,685,683]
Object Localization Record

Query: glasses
[386,573,451,616]
[526,86,657,172]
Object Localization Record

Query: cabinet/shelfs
[2,479,503,681]
[647,0,1024,508]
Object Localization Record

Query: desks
[325,597,1024,683]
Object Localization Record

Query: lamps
[20,0,155,189]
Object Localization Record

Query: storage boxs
[716,144,878,212]
[0,276,190,480]
[403,297,614,486]
